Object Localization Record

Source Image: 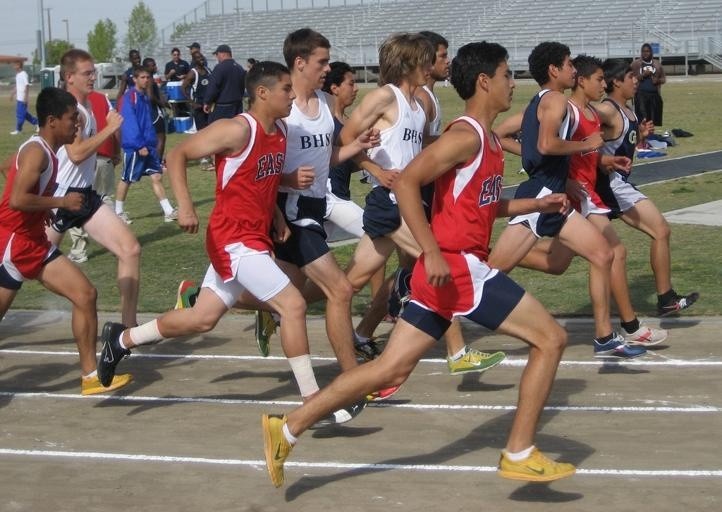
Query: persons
[384,41,648,360]
[0,25,505,428]
[631,44,663,128]
[590,56,702,317]
[262,39,578,492]
[518,53,668,348]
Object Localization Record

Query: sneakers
[639,139,647,148]
[103,196,132,224]
[165,208,179,222]
[255,310,277,357]
[592,327,668,357]
[81,322,134,394]
[354,330,381,360]
[10,130,22,135]
[175,280,200,309]
[658,292,699,316]
[262,414,291,488]
[498,448,576,482]
[34,123,39,133]
[308,385,402,429]
[449,349,505,376]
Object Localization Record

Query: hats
[186,42,200,48]
[213,45,231,55]
